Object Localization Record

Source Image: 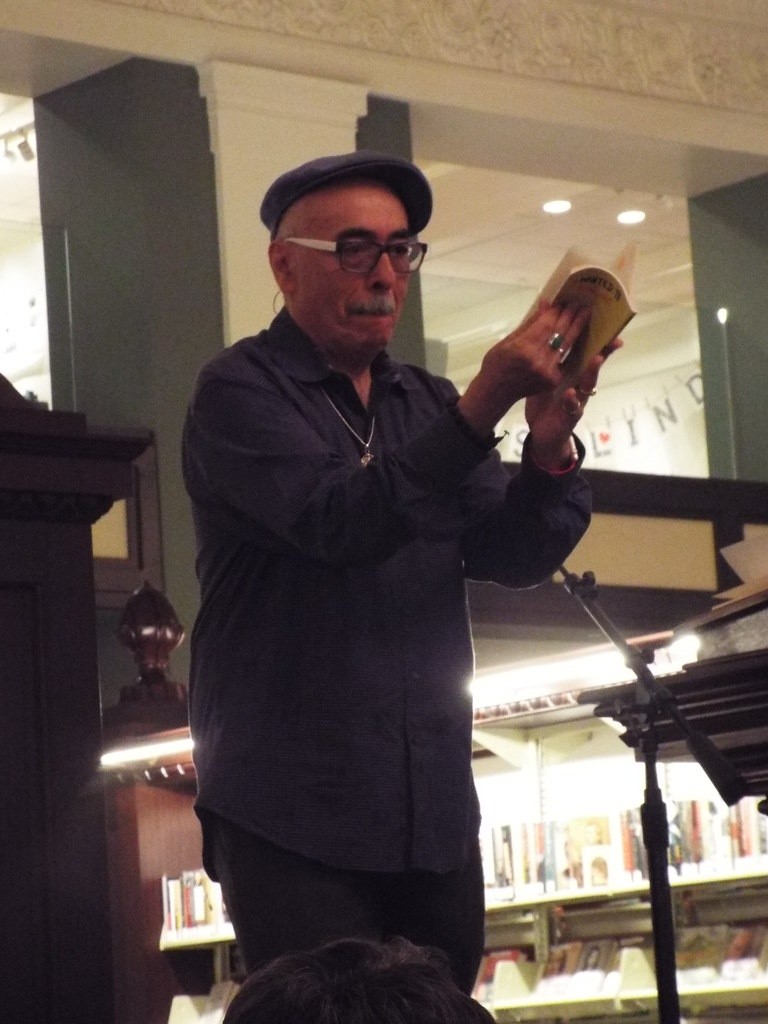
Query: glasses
[284,239,427,277]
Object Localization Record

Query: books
[710,524,768,610]
[524,243,638,398]
[161,867,245,1024]
[467,794,768,1007]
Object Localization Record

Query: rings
[562,402,581,415]
[548,334,563,348]
[559,348,565,354]
[578,388,596,395]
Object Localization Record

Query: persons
[181,154,624,1024]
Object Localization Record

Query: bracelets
[533,455,577,475]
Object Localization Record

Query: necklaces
[320,388,376,471]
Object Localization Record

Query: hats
[259,150,433,241]
